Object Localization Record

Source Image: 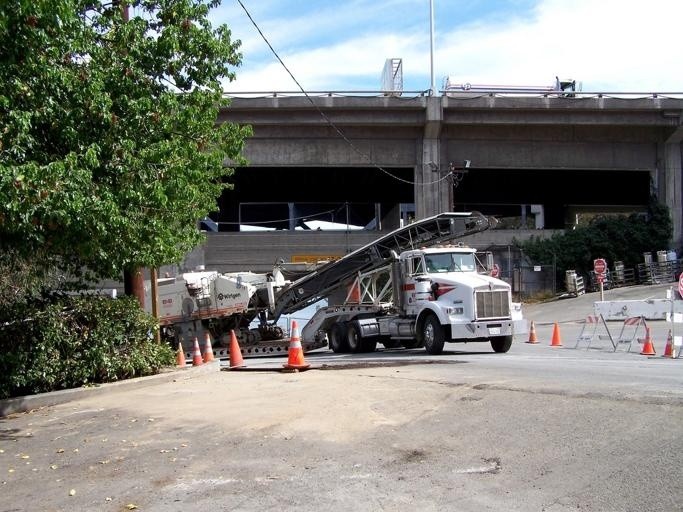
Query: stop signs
[593,259,606,274]
[679,273,683,298]
[492,263,499,276]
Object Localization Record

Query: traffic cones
[175,333,217,368]
[283,319,310,369]
[638,327,674,358]
[225,329,247,369]
[525,319,563,346]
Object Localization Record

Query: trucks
[302,245,528,355]
